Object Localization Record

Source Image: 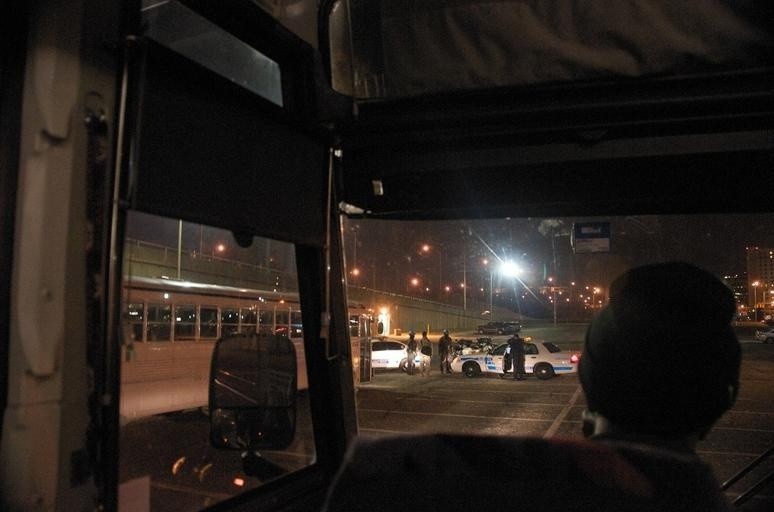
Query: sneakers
[513,377,528,381]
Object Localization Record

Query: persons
[420,331,435,377]
[508,333,527,382]
[438,329,454,376]
[405,330,418,375]
[577,260,742,512]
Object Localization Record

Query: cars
[477,321,513,335]
[449,336,582,380]
[505,322,521,332]
[372,338,432,375]
[755,325,774,344]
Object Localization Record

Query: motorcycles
[454,337,497,356]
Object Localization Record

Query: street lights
[423,245,443,303]
[752,282,760,322]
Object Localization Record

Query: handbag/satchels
[421,346,432,356]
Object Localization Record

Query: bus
[119,274,375,423]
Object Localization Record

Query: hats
[577,261,741,431]
